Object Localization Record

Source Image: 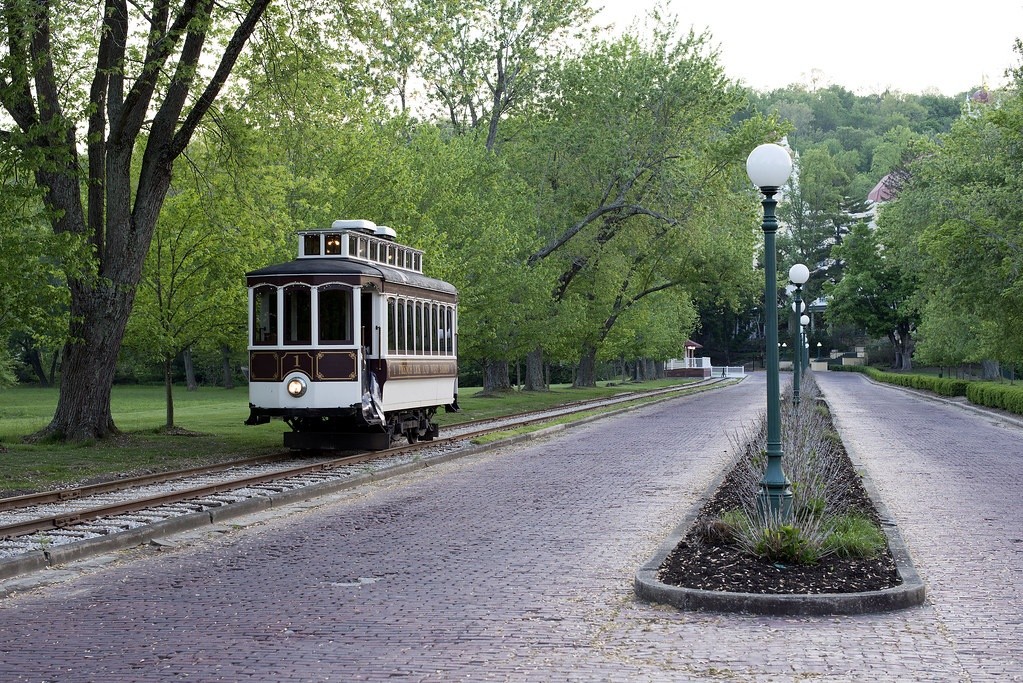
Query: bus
[244,220,460,450]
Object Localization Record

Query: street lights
[790,263,810,411]
[791,297,810,380]
[745,144,793,510]
[817,342,822,360]
